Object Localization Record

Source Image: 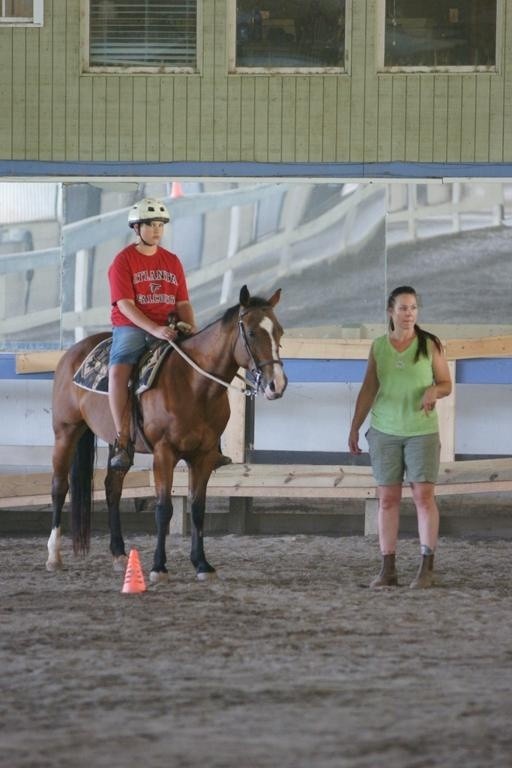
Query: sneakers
[110,448,134,470]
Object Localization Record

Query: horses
[45,284,289,584]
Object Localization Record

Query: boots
[370,554,397,588]
[409,554,434,589]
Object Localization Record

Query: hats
[128,199,170,228]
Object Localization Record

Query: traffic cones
[171,181,184,202]
[117,547,155,596]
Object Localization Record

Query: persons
[348,286,452,590]
[108,198,231,471]
